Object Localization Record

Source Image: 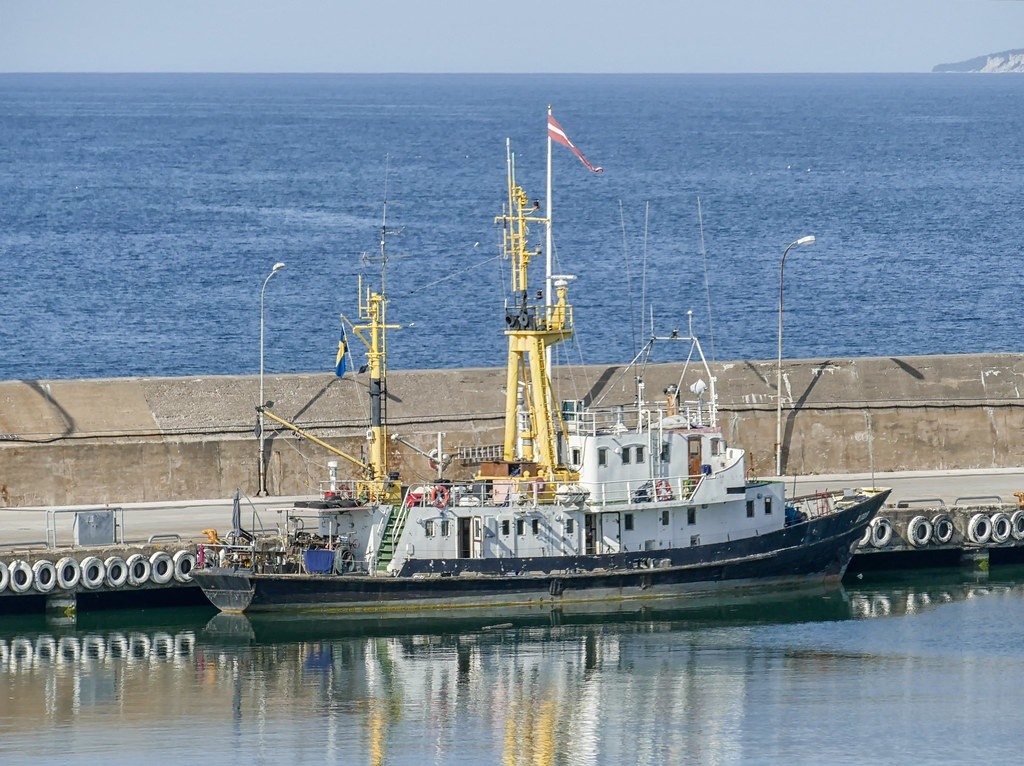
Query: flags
[547,114,604,175]
[334,321,348,379]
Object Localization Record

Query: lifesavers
[431,485,449,508]
[655,481,672,501]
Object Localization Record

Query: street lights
[257,260,285,496]
[775,233,816,476]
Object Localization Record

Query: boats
[191,105,890,641]
